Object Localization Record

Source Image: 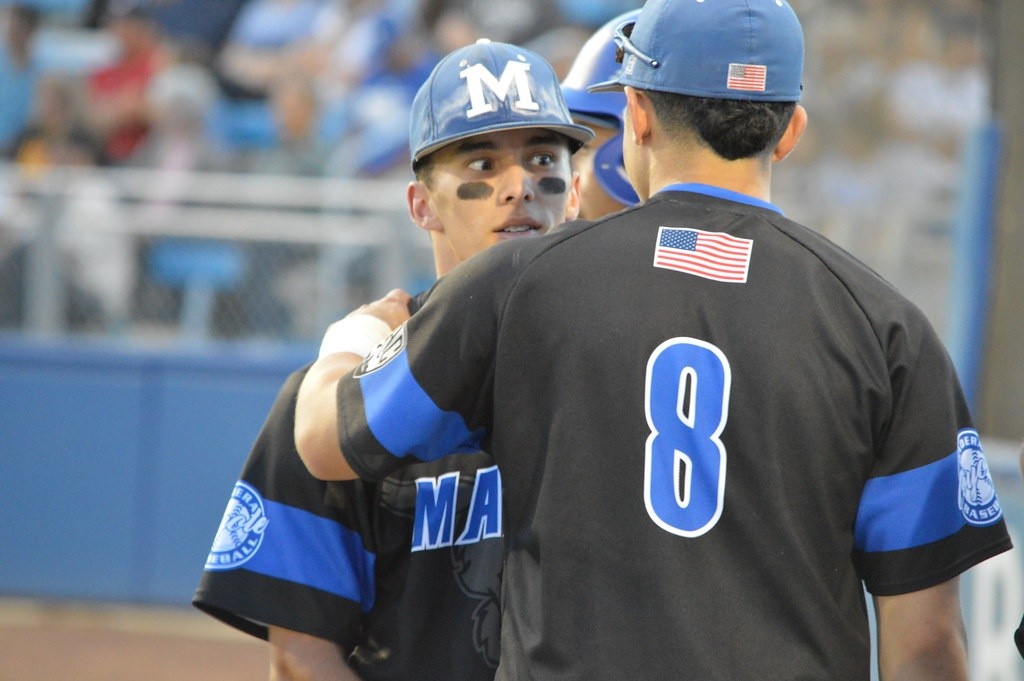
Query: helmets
[559,8,640,206]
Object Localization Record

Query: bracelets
[318,316,393,361]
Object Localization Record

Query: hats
[409,38,595,173]
[585,0,804,102]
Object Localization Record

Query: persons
[190,7,644,680]
[295,0,1014,681]
[0,0,986,346]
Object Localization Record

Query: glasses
[610,20,660,69]
